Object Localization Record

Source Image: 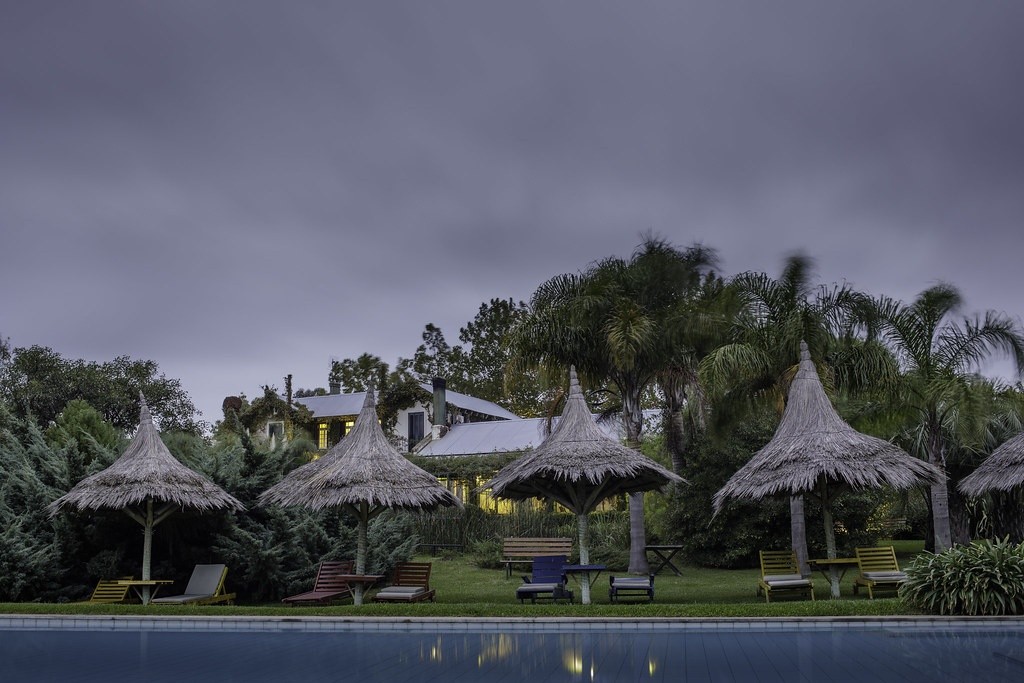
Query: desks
[645,545,686,576]
[117,579,175,603]
[562,564,607,591]
[806,557,861,585]
[336,573,385,600]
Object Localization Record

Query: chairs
[755,550,815,603]
[854,546,904,600]
[150,563,237,606]
[515,554,574,604]
[370,561,435,604]
[609,574,654,602]
[280,560,353,607]
[88,576,134,602]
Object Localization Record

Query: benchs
[498,536,573,579]
